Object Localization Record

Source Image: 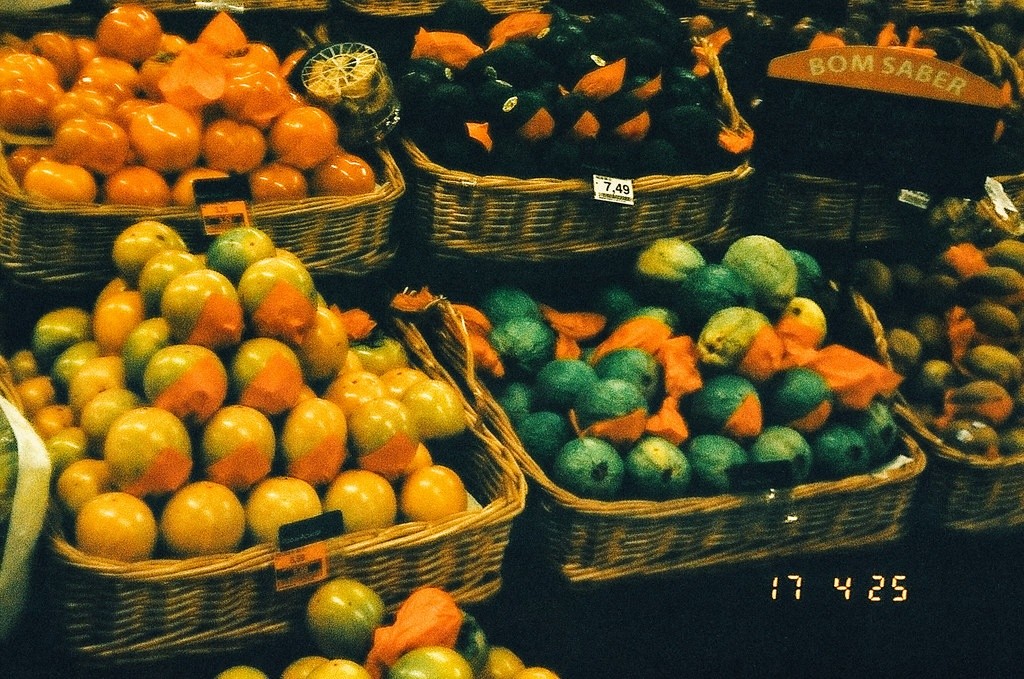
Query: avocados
[488,237,899,496]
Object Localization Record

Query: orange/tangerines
[6,220,560,679]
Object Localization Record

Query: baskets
[0,0,1024,679]
